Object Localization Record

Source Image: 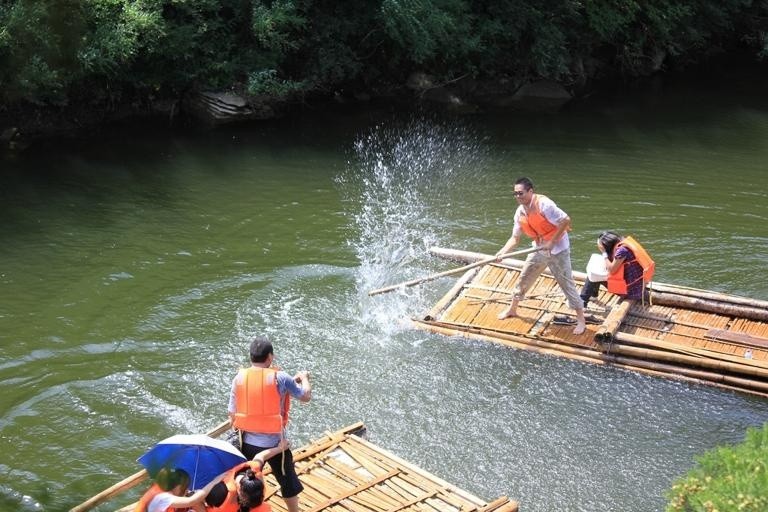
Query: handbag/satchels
[586,253,607,282]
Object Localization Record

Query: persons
[228,341,309,512]
[495,178,586,335]
[580,233,649,305]
[139,438,291,512]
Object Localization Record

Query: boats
[108,422,522,511]
[384,247,768,404]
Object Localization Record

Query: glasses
[512,191,523,195]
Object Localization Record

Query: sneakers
[585,315,603,325]
[553,316,577,325]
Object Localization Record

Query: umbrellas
[137,434,247,496]
